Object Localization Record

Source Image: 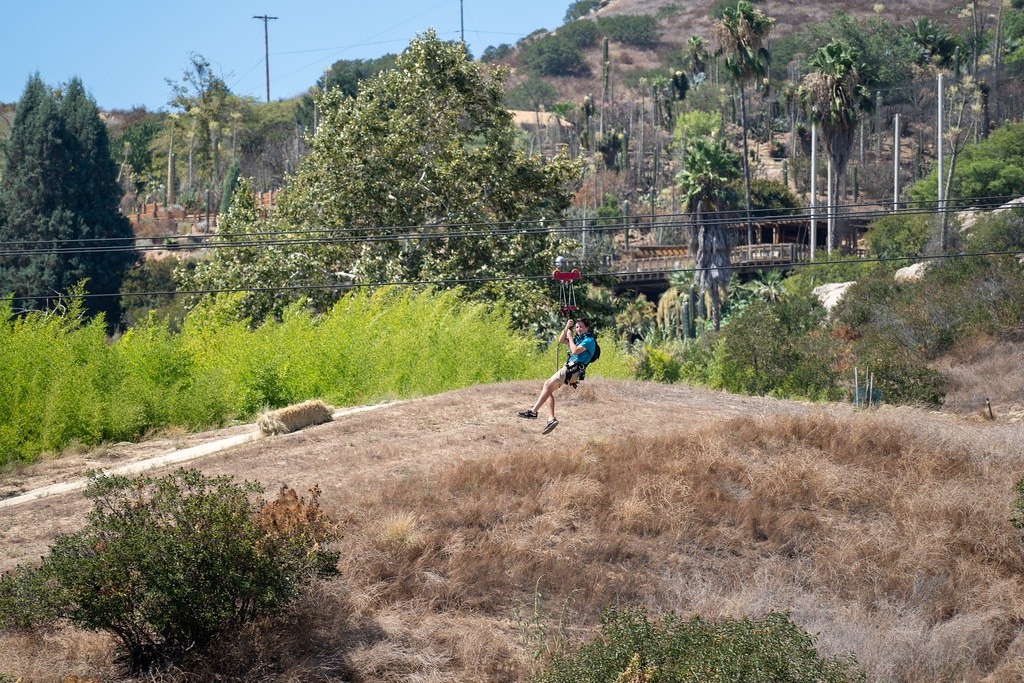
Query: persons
[516,318,596,435]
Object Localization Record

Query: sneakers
[517,410,538,419]
[542,418,559,435]
